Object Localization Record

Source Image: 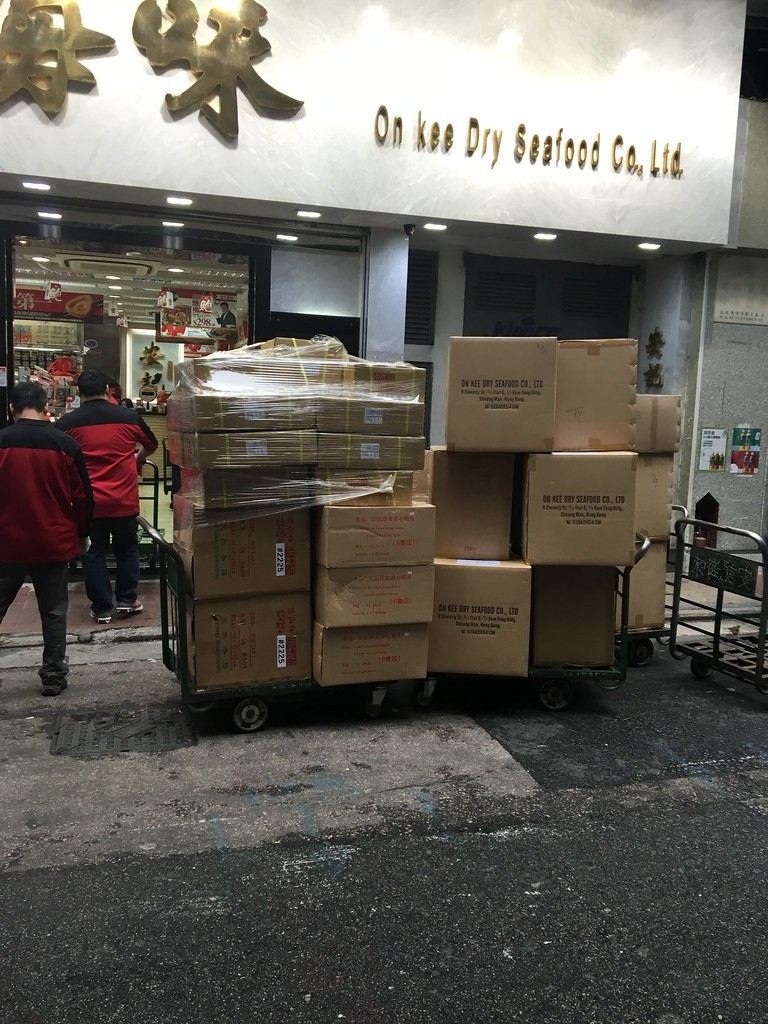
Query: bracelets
[136,454,146,465]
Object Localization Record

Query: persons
[119,398,133,408]
[108,384,119,406]
[0,381,95,697]
[214,301,236,328]
[55,368,158,624]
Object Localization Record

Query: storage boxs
[158,329,686,698]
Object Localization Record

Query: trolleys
[134,513,400,733]
[669,518,768,694]
[67,459,160,573]
[408,503,690,713]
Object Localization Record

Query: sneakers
[42,679,67,696]
[90,610,111,623]
[116,599,144,613]
